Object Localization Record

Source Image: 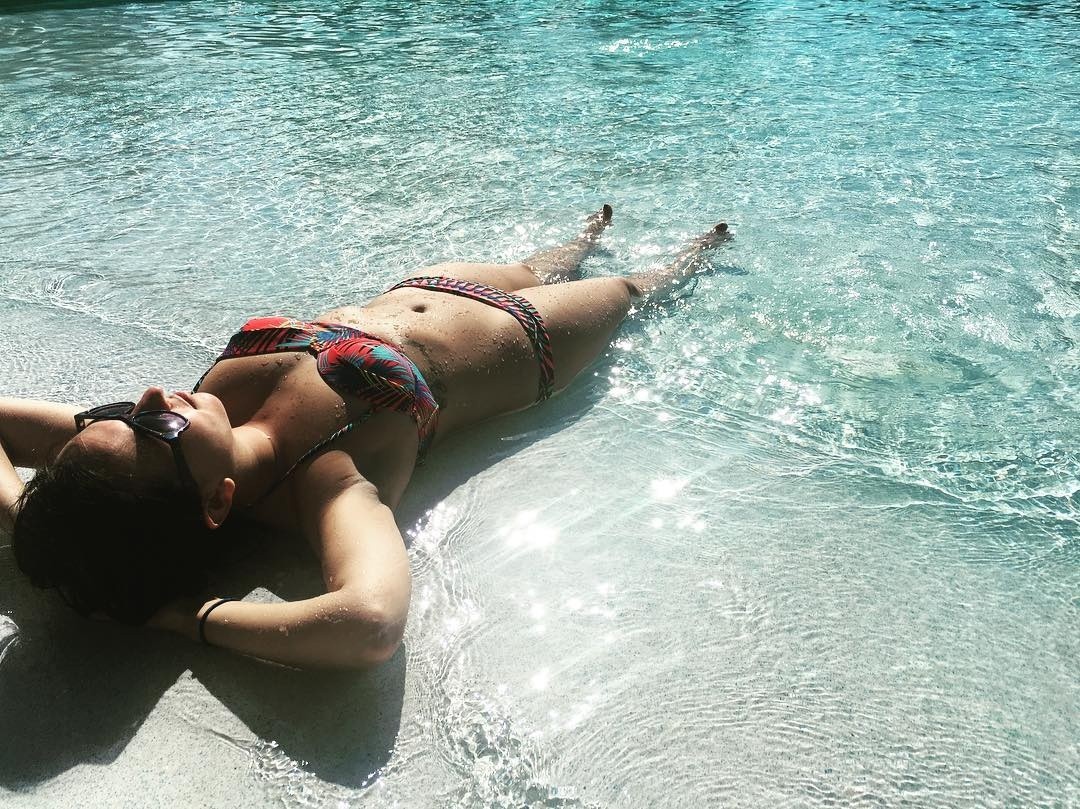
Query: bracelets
[199,597,239,645]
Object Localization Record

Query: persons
[0,204,728,673]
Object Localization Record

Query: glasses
[73,401,201,504]
